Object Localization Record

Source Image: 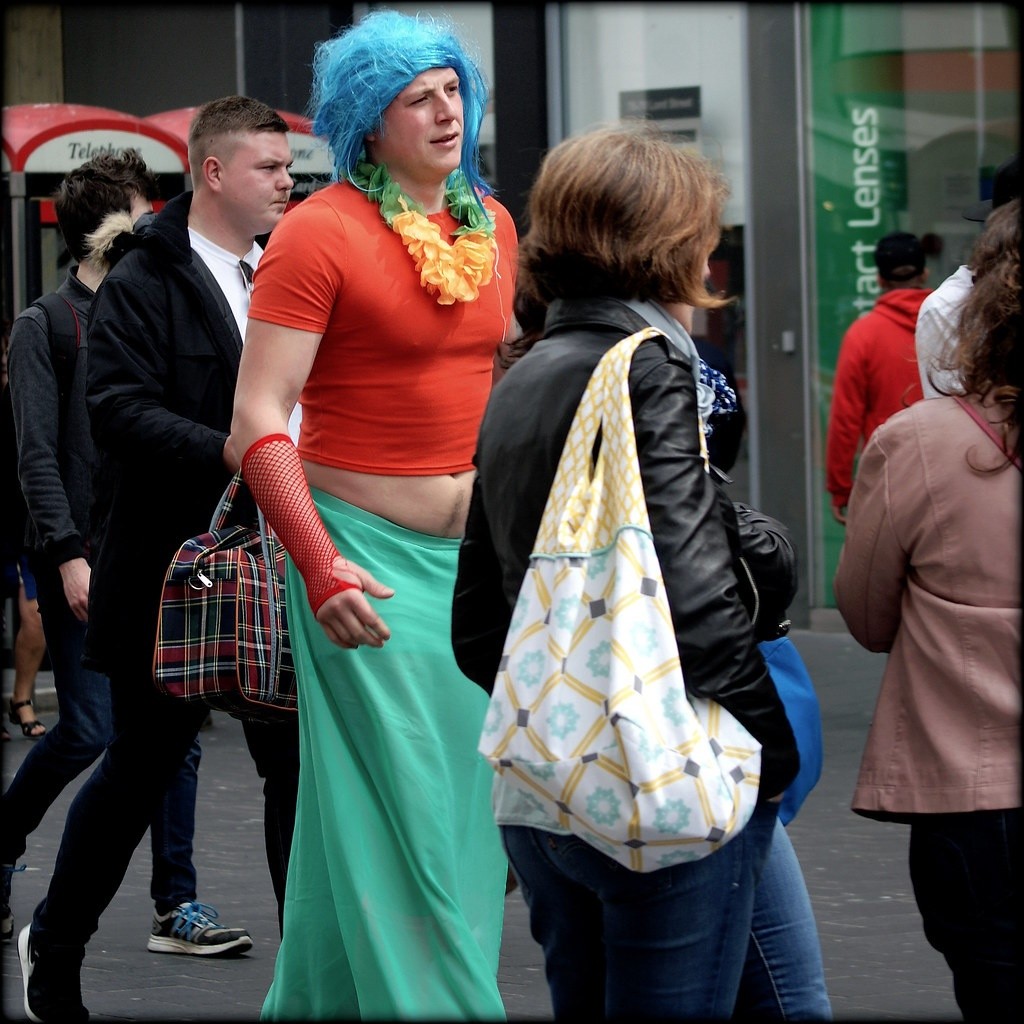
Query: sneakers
[144,900,254,956]
[0,861,29,942]
[18,920,89,1024]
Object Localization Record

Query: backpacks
[0,292,82,562]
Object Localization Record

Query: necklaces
[356,155,496,304]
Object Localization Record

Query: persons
[916,200,1024,404]
[834,268,1024,1022]
[451,132,831,1024]
[734,829,830,1023]
[223,10,518,1021]
[1,95,299,1024]
[824,233,940,526]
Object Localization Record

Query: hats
[873,232,927,282]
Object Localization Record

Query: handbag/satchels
[151,467,300,713]
[471,328,765,875]
[753,633,825,828]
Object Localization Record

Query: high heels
[9,696,48,737]
